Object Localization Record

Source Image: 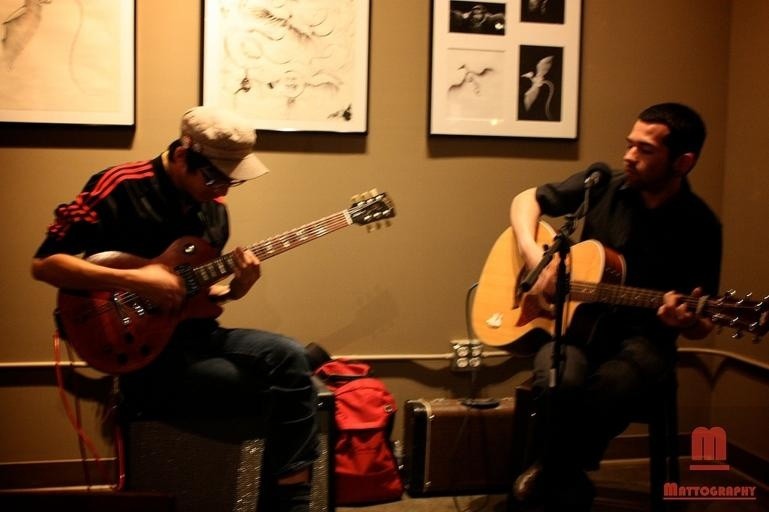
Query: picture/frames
[0,1,136,128]
[199,1,372,138]
[427,1,587,143]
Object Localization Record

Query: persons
[31,107,320,512]
[510,102,721,511]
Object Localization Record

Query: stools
[127,422,331,512]
[512,376,682,510]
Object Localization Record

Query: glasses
[196,166,246,188]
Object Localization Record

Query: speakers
[118,391,335,512]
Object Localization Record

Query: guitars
[52,189,396,375]
[470,221,769,352]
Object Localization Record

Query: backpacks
[304,343,404,501]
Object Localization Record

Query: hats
[182,108,269,181]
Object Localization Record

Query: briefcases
[404,396,516,498]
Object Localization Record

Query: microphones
[584,162,610,189]
